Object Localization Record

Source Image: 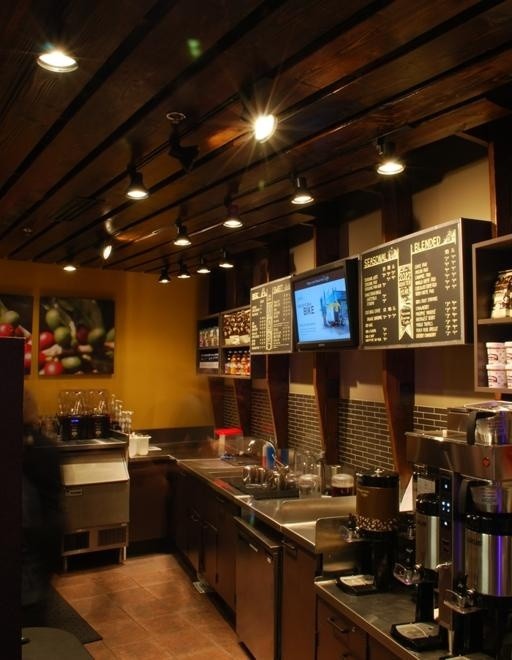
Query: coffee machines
[333,465,414,595]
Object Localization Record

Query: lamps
[37,35,78,72]
[93,164,319,285]
[369,133,411,177]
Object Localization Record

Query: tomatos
[0,323,64,375]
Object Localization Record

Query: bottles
[224,349,251,376]
[485,341,512,389]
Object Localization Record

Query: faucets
[246,437,291,490]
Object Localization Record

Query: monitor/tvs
[290,258,359,350]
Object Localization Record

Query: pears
[45,308,115,370]
[0,311,20,323]
